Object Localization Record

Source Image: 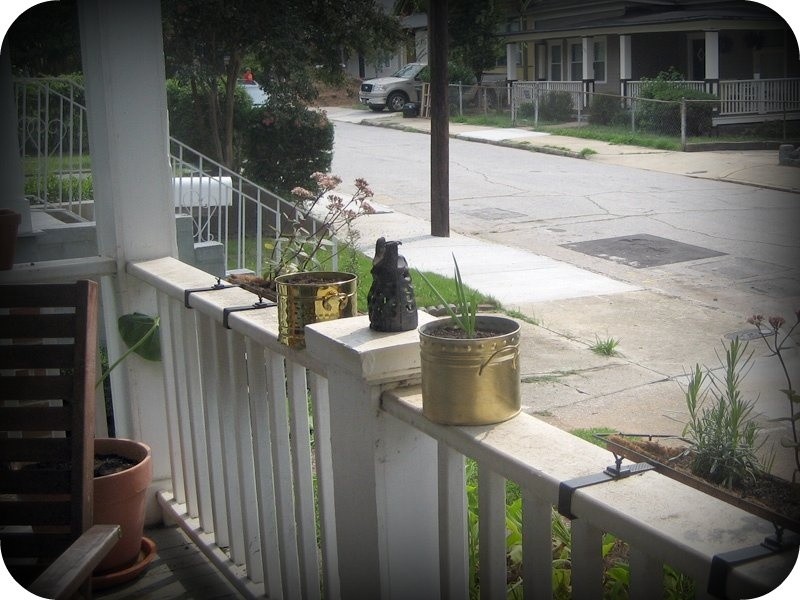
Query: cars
[238,79,272,108]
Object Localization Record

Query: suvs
[358,62,499,113]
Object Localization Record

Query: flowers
[261,172,380,286]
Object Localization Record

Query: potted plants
[610,336,800,535]
[88,309,164,587]
[415,249,524,429]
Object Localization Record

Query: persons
[244,67,252,82]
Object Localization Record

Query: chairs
[0,279,122,600]
